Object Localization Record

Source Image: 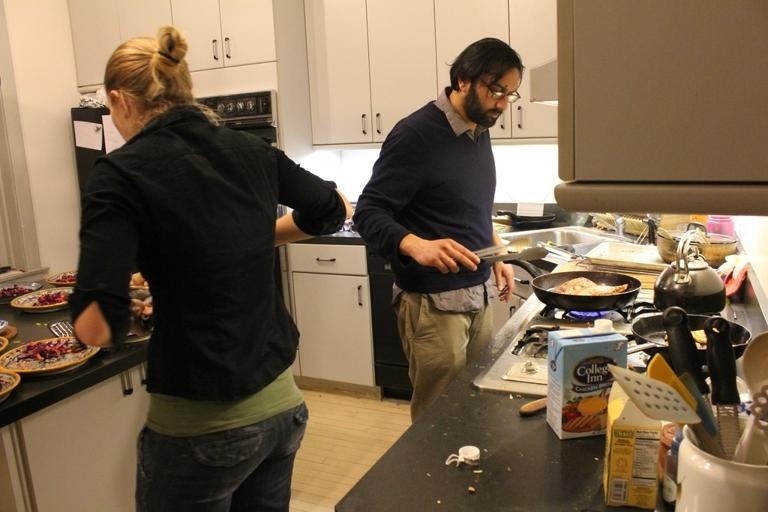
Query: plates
[0,269,101,405]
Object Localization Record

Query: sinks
[550,238,638,259]
[499,225,606,248]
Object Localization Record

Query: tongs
[476,236,549,265]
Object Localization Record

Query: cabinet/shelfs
[484,263,551,342]
[279,244,382,402]
[555,0,768,215]
[304,0,437,146]
[66,2,173,92]
[433,0,560,145]
[190,61,315,162]
[171,1,309,74]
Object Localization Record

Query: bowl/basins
[655,229,739,265]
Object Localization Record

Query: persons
[68,26,347,512]
[352,38,524,423]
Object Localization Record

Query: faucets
[588,212,625,236]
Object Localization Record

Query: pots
[625,312,750,357]
[493,210,555,227]
[504,260,642,312]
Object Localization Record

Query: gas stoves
[475,309,626,393]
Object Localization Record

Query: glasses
[480,78,520,103]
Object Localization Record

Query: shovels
[607,363,727,460]
[48,319,76,338]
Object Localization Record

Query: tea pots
[655,230,727,315]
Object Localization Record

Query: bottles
[709,216,733,235]
[664,419,768,512]
